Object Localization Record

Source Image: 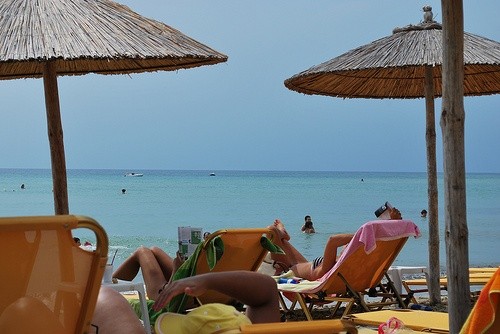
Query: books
[178,225,204,259]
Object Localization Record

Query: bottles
[278,277,298,284]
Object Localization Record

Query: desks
[387,265,433,304]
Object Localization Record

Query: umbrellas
[284,5,500,305]
[0,0,228,215]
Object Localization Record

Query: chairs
[0,215,500,334]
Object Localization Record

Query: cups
[103,264,114,282]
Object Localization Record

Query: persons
[122,189,126,194]
[0,207,402,334]
[21,184,25,189]
[420,210,428,218]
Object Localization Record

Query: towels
[459,266,499,332]
[123,232,287,321]
[272,218,423,294]
